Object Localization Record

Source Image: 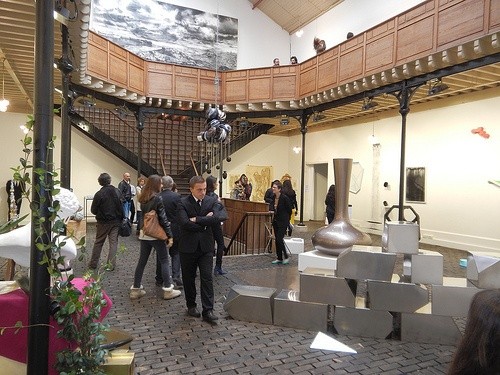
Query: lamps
[312,109,327,124]
[428,76,449,96]
[280,115,289,127]
[198,13,232,181]
[362,95,379,111]
[240,119,249,128]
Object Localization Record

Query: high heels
[214,266,227,275]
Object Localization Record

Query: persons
[6,173,22,227]
[291,56,297,64]
[119,173,144,230]
[270,181,292,264]
[206,176,228,275]
[89,173,124,271]
[156,176,182,288]
[347,32,353,39]
[274,58,279,66]
[325,185,335,224]
[235,174,252,200]
[264,180,298,236]
[449,288,500,375]
[130,175,181,298]
[177,176,218,319]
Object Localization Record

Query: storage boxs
[101,348,136,375]
[67,219,86,250]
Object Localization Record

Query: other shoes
[282,257,290,265]
[273,259,282,264]
[202,311,219,320]
[130,285,146,298]
[188,308,201,317]
[162,284,181,299]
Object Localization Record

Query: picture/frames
[404,166,426,205]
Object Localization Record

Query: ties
[197,200,201,207]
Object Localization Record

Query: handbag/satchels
[218,203,230,221]
[144,209,171,241]
[120,218,131,236]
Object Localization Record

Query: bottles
[313,159,373,254]
